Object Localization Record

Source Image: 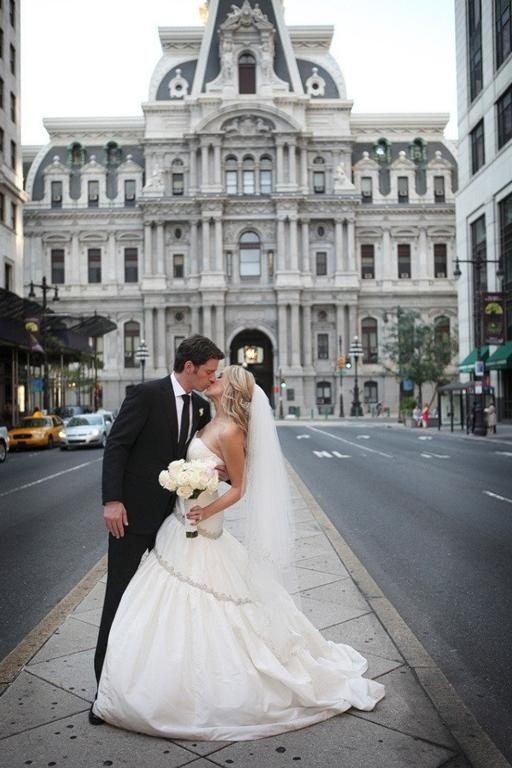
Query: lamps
[373,138,387,156]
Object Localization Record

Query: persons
[421,403,428,428]
[89,365,258,743]
[484,400,496,432]
[87,331,227,724]
[413,405,422,427]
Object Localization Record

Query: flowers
[159,458,222,537]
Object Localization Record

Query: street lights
[452,247,505,435]
[348,336,364,416]
[28,276,60,415]
[135,339,150,382]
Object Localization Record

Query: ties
[179,394,192,448]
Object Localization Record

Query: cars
[0,406,116,463]
